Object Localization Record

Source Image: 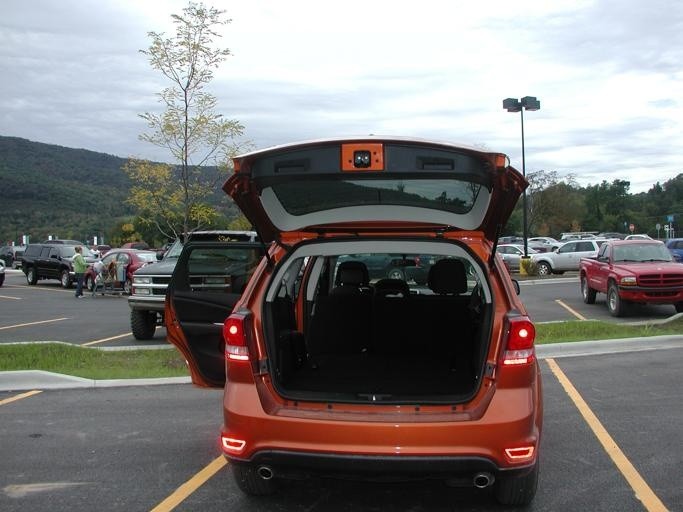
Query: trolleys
[88,259,122,299]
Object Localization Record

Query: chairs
[424,256,469,297]
[329,260,373,294]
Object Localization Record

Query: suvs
[162,133,544,510]
[126,231,256,343]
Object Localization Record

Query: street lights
[498,95,545,273]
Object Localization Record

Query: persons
[90,245,100,256]
[92,254,108,296]
[72,246,90,299]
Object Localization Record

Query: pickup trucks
[577,238,682,317]
